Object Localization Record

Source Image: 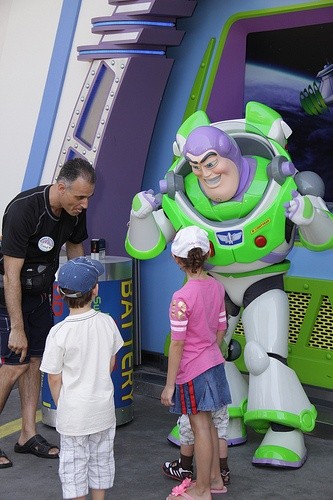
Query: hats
[58,257,105,297]
[171,226,210,258]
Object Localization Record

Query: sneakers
[221,469,230,484]
[162,459,193,482]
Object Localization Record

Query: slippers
[181,478,227,493]
[0,449,13,468]
[15,434,60,458]
[166,485,195,500]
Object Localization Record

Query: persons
[40,257,125,500]
[0,157,96,468]
[160,227,230,499]
[125,101,333,466]
